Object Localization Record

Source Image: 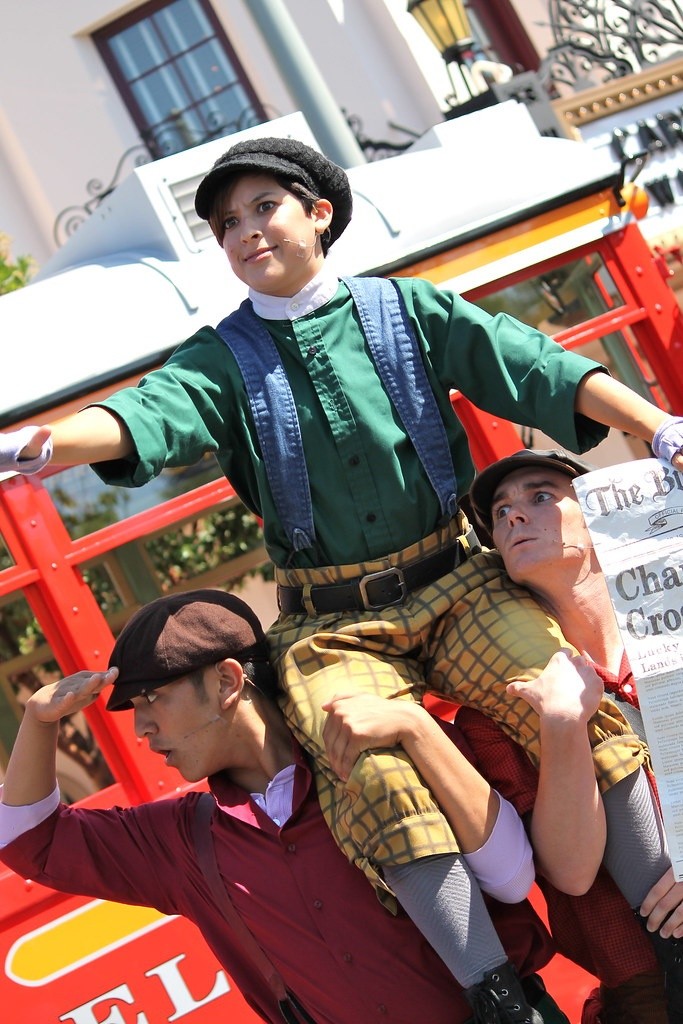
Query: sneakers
[463,964,571,1024]
[630,897,683,1024]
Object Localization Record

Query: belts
[278,523,483,617]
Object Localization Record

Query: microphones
[553,540,594,549]
[183,715,220,739]
[284,235,318,247]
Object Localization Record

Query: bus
[0,111,683,1024]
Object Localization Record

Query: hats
[469,450,601,521]
[194,137,353,251]
[106,588,267,711]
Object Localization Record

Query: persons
[0,590,570,1024]
[452,447,683,1024]
[0,137,683,1024]
